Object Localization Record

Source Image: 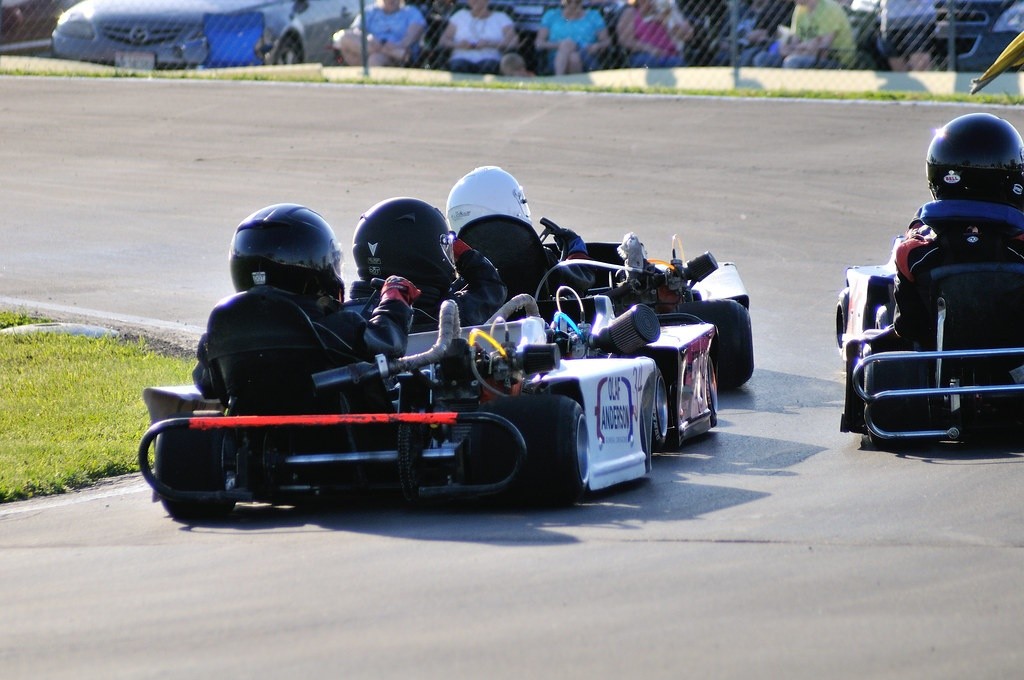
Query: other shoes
[499,52,527,77]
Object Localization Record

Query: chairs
[207,288,358,414]
[172,12,272,69]
[907,241,1024,350]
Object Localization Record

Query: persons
[736,0,856,68]
[333,0,426,66]
[438,0,518,74]
[350,198,506,327]
[448,167,595,296]
[535,0,612,73]
[893,113,1024,338]
[191,205,419,409]
[880,0,938,69]
[618,0,693,67]
[499,53,533,77]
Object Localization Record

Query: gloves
[905,222,931,242]
[440,231,471,263]
[552,228,589,256]
[380,275,422,310]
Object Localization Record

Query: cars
[52,0,376,71]
[839,0,1024,72]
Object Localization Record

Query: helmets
[352,196,457,298]
[445,165,532,237]
[228,203,343,302]
[925,111,1024,210]
